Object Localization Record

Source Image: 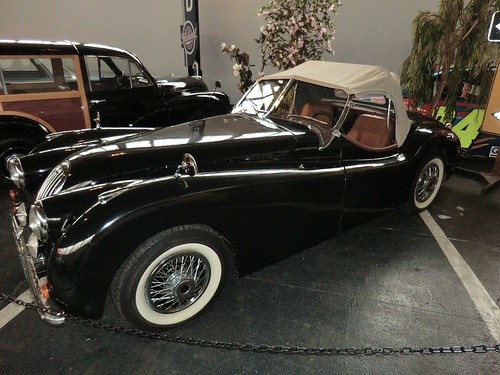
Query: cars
[0,39,230,190]
[5,60,462,332]
[358,66,500,163]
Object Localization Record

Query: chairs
[299,101,335,128]
[345,114,394,149]
